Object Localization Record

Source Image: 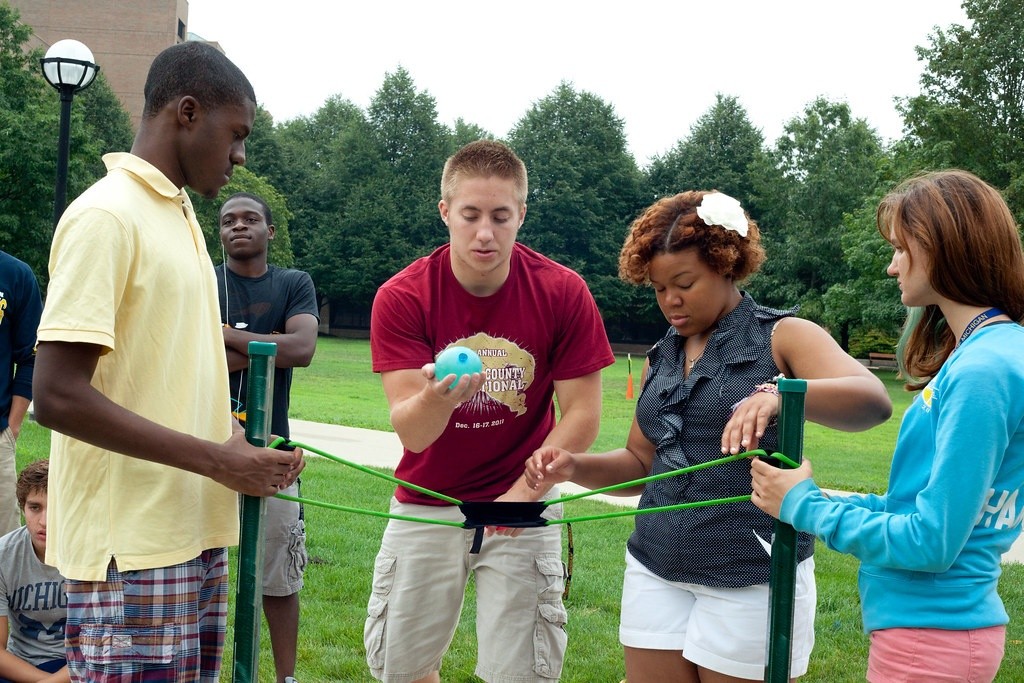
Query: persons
[215,192,321,683]
[0,459,71,683]
[524,190,892,683]
[363,139,615,683]
[33,42,305,682]
[0,251,42,530]
[750,168,1024,683]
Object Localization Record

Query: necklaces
[684,345,705,368]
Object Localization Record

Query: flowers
[696,192,749,238]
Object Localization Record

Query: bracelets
[731,373,790,412]
